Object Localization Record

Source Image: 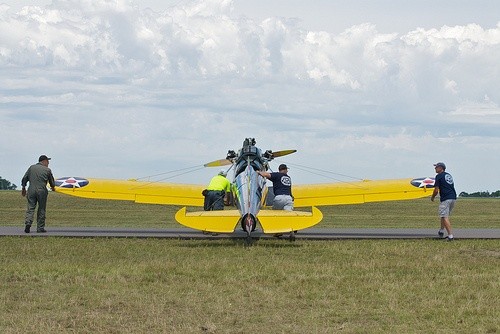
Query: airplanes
[49,138,440,242]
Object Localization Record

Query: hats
[39,155,51,162]
[279,164,290,170]
[433,162,446,169]
[218,170,227,177]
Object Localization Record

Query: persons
[256,164,295,242]
[20,155,56,233]
[203,170,231,236]
[431,162,457,242]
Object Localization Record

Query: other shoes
[37,229,47,233]
[289,235,295,241]
[25,225,30,233]
[212,232,218,236]
[203,231,209,234]
[445,236,453,241]
[273,233,282,237]
[438,231,444,237]
